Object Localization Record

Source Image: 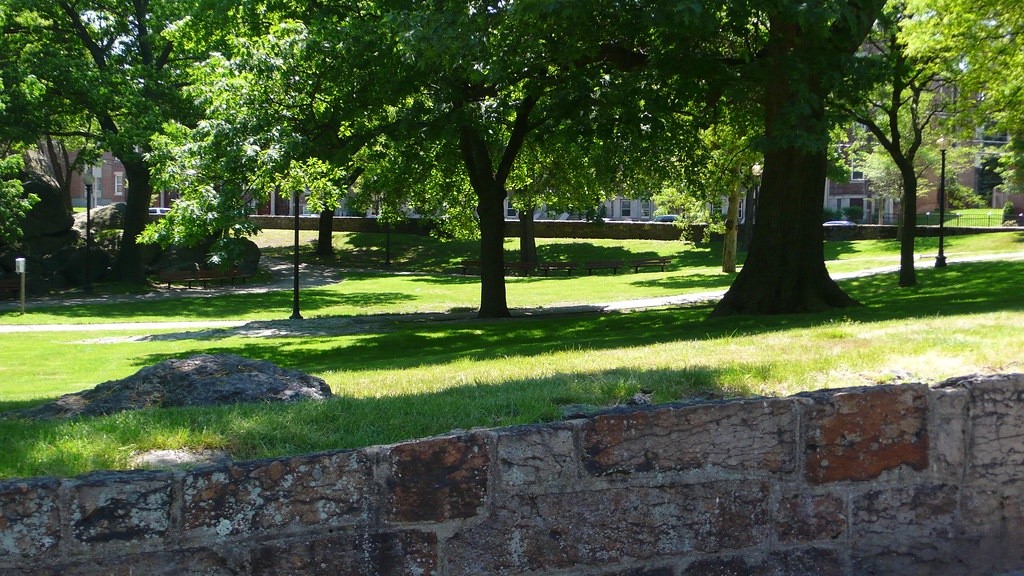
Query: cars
[650,215,678,222]
[609,216,640,221]
[823,221,856,225]
[149,208,172,215]
[582,217,613,221]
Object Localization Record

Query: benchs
[197,270,229,289]
[504,262,537,276]
[631,259,671,273]
[585,261,624,276]
[456,261,481,274]
[538,261,581,276]
[157,270,196,290]
[226,267,256,285]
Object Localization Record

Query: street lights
[751,165,763,208]
[935,138,948,267]
[82,173,95,289]
